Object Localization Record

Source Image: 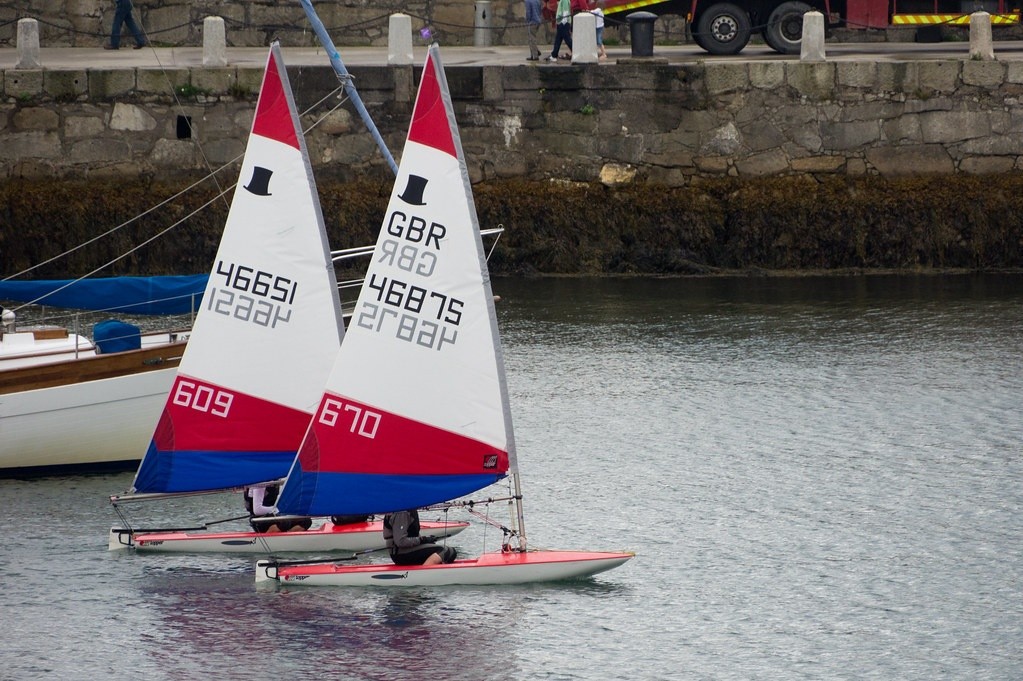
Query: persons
[545,0,575,61]
[582,0,608,60]
[525,0,543,60]
[243,478,313,533]
[383,509,458,564]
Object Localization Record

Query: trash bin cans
[626,11,658,56]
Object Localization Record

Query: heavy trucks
[548,1,1023,57]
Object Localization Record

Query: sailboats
[243,40,637,588]
[103,40,472,557]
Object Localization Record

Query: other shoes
[526,50,571,61]
[599,54,607,60]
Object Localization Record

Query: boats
[2,219,507,475]
